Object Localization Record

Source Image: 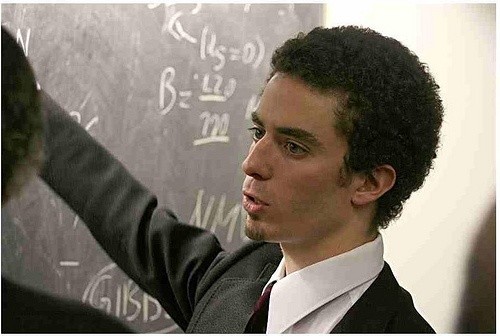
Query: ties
[248,281,276,334]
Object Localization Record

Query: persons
[13,24,445,334]
[1,25,140,334]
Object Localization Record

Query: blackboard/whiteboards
[3,5,324,334]
